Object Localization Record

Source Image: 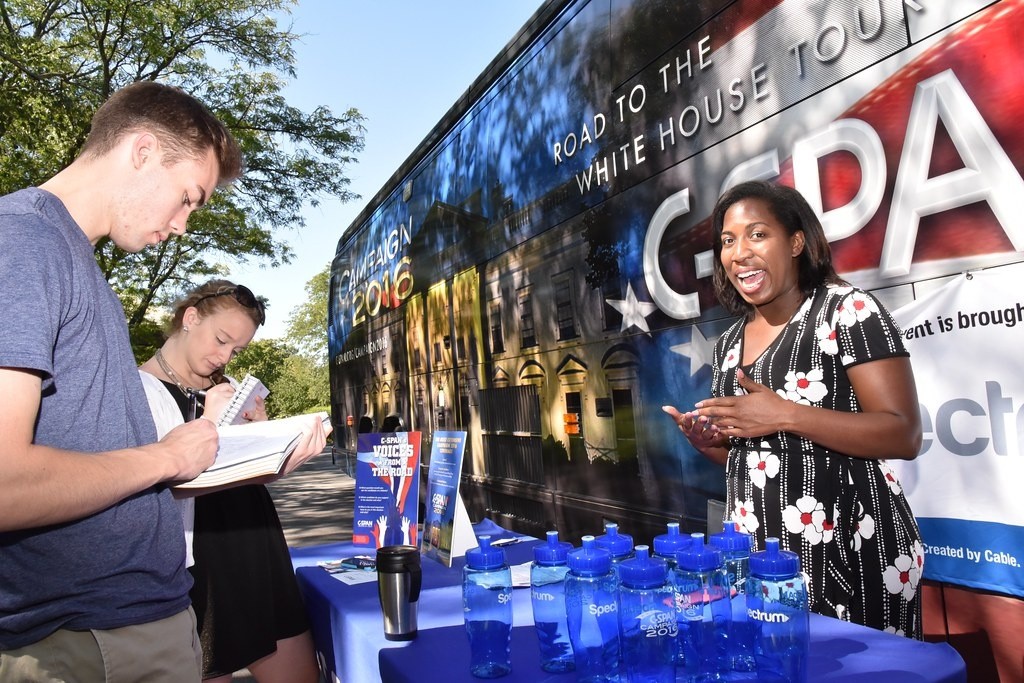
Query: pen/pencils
[185,386,208,396]
[189,395,200,424]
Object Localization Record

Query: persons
[0,78,243,683]
[658,178,926,646]
[133,278,323,683]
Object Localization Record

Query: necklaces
[154,348,207,413]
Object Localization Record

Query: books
[218,373,269,427]
[167,410,337,501]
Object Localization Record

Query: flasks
[377,543,423,642]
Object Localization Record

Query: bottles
[706,523,763,673]
[566,534,623,683]
[592,521,638,679]
[530,531,578,673]
[675,532,733,683]
[653,523,706,667]
[618,545,678,683]
[461,533,516,679]
[744,536,811,683]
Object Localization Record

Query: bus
[326,2,1024,680]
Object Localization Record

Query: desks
[286,517,970,682]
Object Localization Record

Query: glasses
[194,285,265,327]
